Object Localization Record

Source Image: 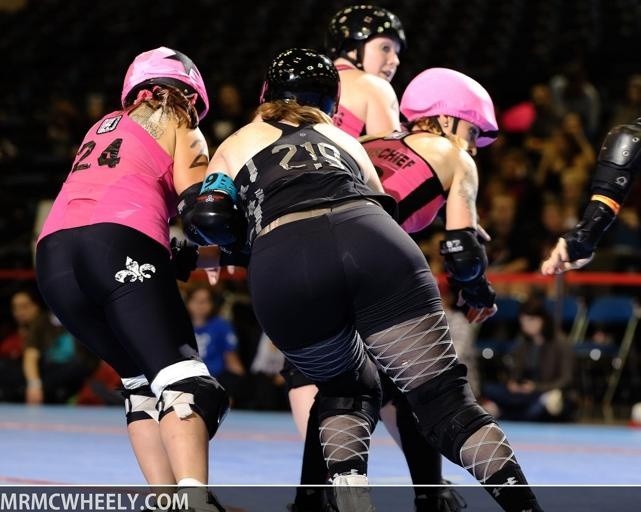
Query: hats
[323,4,410,61]
[397,66,502,149]
[119,45,209,123]
[258,47,341,123]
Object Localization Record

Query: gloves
[557,198,617,268]
[461,278,497,310]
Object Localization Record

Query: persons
[35,44,232,510]
[0,73,639,424]
[199,45,543,510]
[279,4,420,450]
[285,65,507,512]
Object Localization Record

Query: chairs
[484,290,639,421]
